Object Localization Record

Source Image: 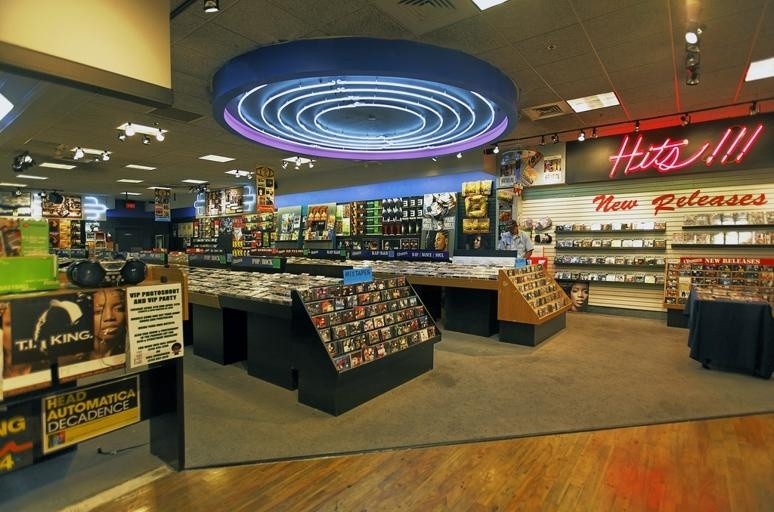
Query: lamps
[71,119,169,163]
[455,97,774,160]
[212,37,518,161]
[683,26,703,87]
[235,153,319,180]
[203,0,220,13]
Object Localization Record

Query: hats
[504,219,516,229]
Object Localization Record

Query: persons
[498,220,534,260]
[259,196,265,205]
[434,231,448,251]
[545,160,560,171]
[2,309,45,380]
[303,279,434,371]
[567,282,588,312]
[385,241,393,251]
[171,343,182,355]
[77,290,126,362]
[472,234,484,249]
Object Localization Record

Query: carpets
[0,307,774,472]
